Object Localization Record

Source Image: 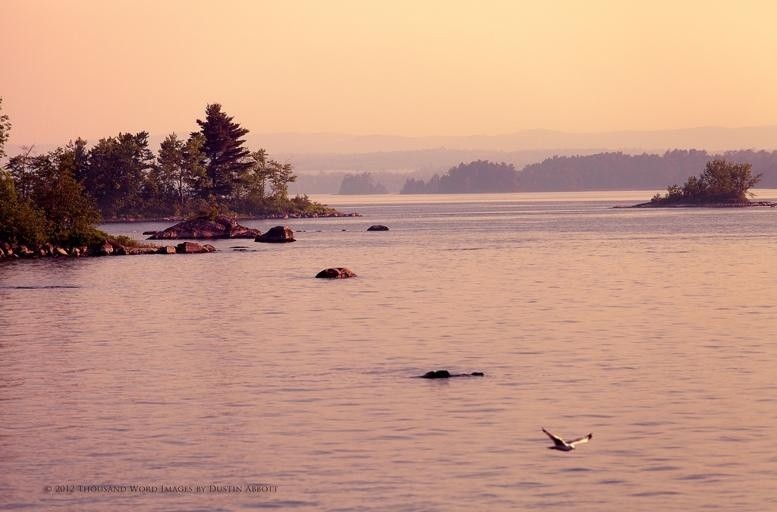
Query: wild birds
[541,427,592,452]
